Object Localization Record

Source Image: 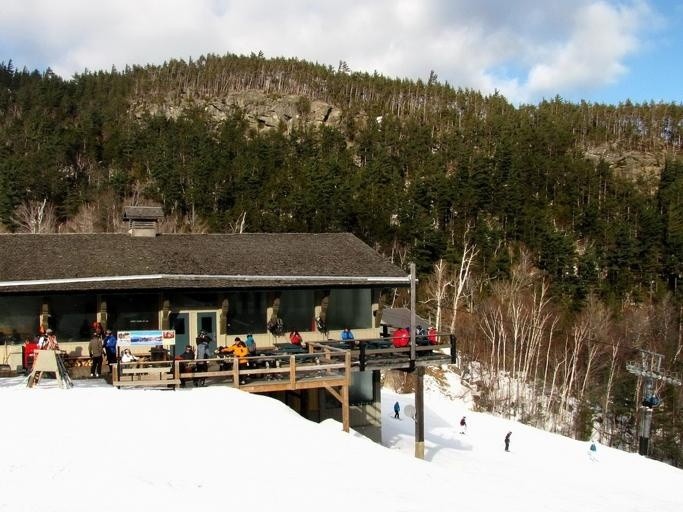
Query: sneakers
[91,373,99,377]
[194,383,207,387]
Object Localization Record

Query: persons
[504,431,513,452]
[640,393,650,408]
[393,401,401,419]
[647,393,659,409]
[459,416,468,435]
[588,440,598,460]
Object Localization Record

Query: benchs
[250,338,393,363]
[114,368,181,390]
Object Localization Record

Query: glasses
[125,352,130,354]
